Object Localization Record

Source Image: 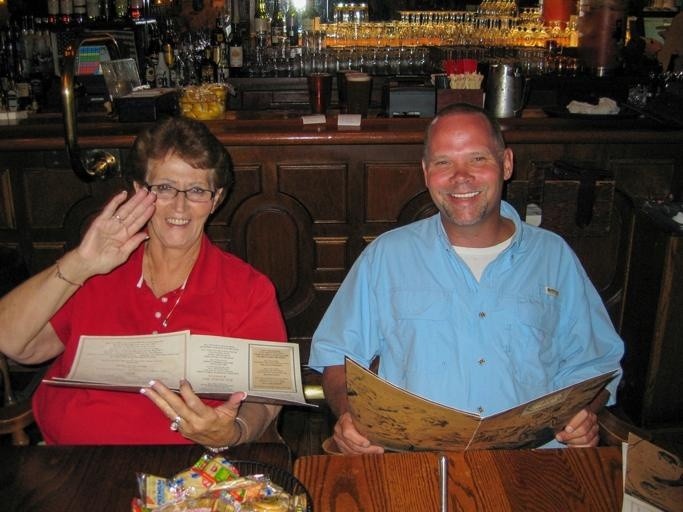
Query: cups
[301,30,326,57]
[307,70,371,119]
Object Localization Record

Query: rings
[174,415,181,424]
[170,423,178,431]
[115,214,122,222]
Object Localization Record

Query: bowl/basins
[176,90,227,121]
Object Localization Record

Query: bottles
[0,0,298,106]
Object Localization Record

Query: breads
[179,84,225,120]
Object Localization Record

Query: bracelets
[203,422,242,453]
[54,259,81,287]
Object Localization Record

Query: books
[40,329,320,410]
[344,356,620,450]
[621,428,683,512]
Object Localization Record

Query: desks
[2,437,622,512]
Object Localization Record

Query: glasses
[140,183,217,204]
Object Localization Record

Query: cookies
[132,456,308,512]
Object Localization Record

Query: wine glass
[299,50,429,69]
[328,0,578,51]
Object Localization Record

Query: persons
[306,103,624,456]
[1,115,289,453]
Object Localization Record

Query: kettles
[486,63,534,119]
